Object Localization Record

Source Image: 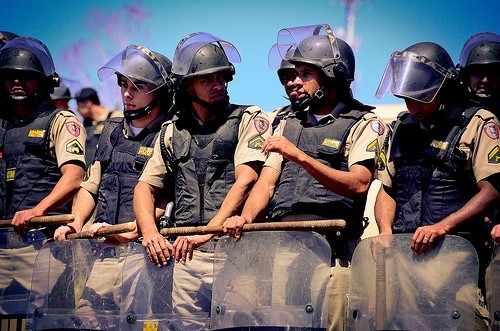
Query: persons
[221,23,390,331]
[454,31,500,109]
[268,38,300,124]
[132,31,272,331]
[53,44,174,331]
[0,35,86,321]
[51,84,73,109]
[74,85,125,164]
[374,42,500,331]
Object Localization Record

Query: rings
[423,237,429,241]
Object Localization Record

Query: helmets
[49,85,71,100]
[390,42,460,99]
[456,40,500,79]
[171,42,236,93]
[0,45,60,92]
[114,50,176,97]
[288,35,355,83]
[278,50,299,85]
